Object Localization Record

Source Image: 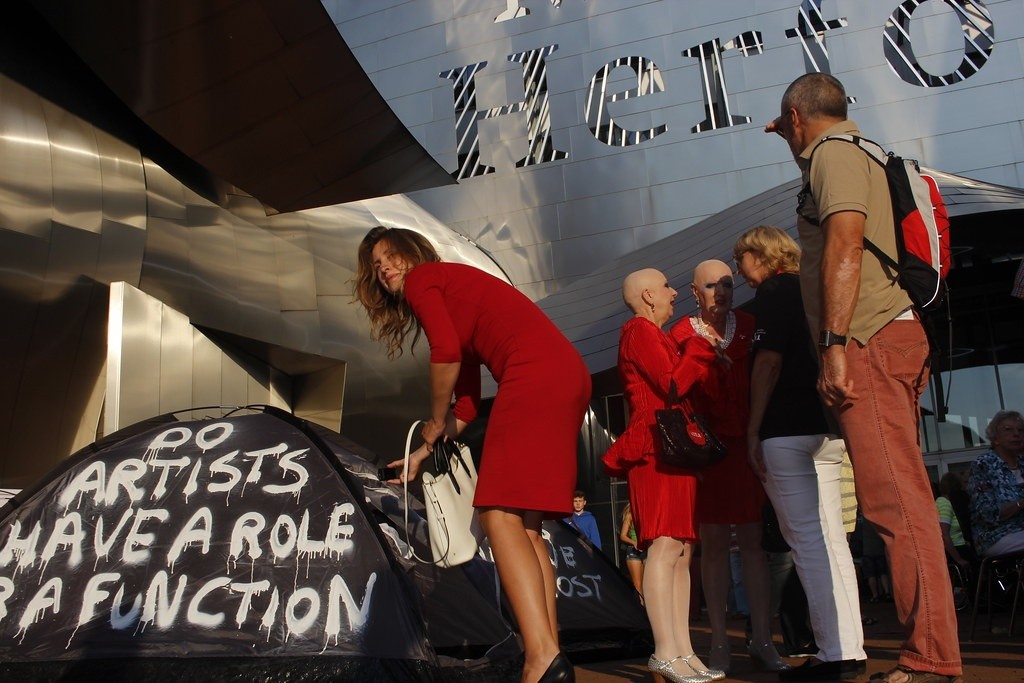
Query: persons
[935,472,1006,612]
[764,72,963,683]
[601,267,733,683]
[733,224,868,681]
[563,491,601,551]
[351,225,593,683]
[667,259,791,675]
[967,412,1024,557]
[620,502,644,607]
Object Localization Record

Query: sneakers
[778,656,857,682]
[855,661,867,676]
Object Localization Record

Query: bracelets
[1016,501,1022,508]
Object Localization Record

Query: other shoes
[884,590,891,601]
[867,664,962,683]
[870,592,879,603]
[790,639,819,658]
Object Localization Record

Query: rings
[715,338,718,342]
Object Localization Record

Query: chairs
[949,488,1024,644]
[947,564,973,614]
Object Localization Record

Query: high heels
[684,652,725,680]
[749,642,791,672]
[538,652,575,683]
[647,654,712,683]
[710,641,731,673]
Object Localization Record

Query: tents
[0,403,655,683]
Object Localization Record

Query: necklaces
[690,310,736,349]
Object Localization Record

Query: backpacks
[797,134,950,309]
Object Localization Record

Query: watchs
[818,330,847,347]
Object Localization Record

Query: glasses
[998,426,1024,434]
[774,107,801,140]
[732,250,752,267]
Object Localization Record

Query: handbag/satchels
[656,378,728,469]
[404,420,488,569]
[954,563,973,614]
[762,496,791,555]
